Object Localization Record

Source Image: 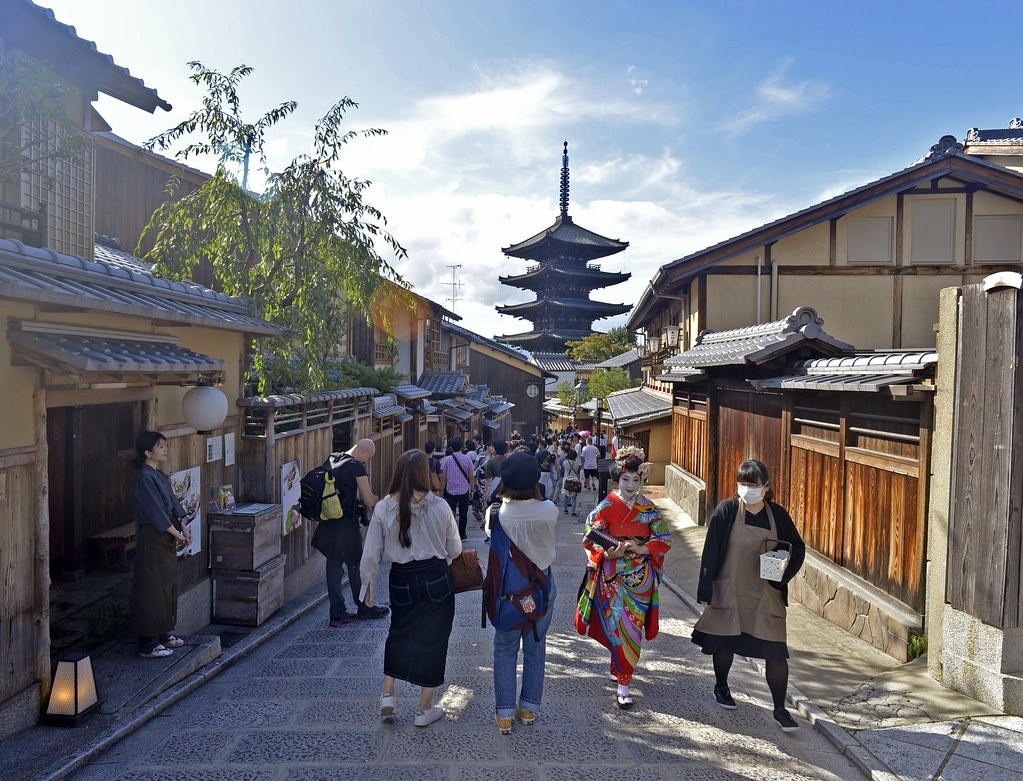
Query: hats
[500,452,541,491]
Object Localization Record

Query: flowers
[615,445,654,475]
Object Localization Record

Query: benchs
[90,521,136,573]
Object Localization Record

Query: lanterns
[181,381,230,436]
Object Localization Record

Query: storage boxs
[208,502,287,627]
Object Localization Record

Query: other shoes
[564,510,568,514]
[473,512,482,520]
[617,691,633,709]
[461,535,468,541]
[571,513,577,516]
[592,484,596,491]
[484,536,491,542]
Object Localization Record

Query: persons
[443,434,534,544]
[359,450,461,728]
[310,439,391,626]
[481,452,559,736]
[526,422,609,504]
[574,445,671,710]
[424,441,443,497]
[580,438,601,491]
[440,437,475,542]
[562,449,579,515]
[131,430,191,657]
[691,459,806,733]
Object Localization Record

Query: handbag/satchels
[564,480,582,493]
[469,488,474,501]
[450,549,483,594]
[480,500,551,642]
[430,458,441,491]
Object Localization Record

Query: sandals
[162,635,184,647]
[140,646,172,657]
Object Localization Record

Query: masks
[738,481,768,505]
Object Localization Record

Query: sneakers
[773,708,799,732]
[516,708,536,726]
[329,610,357,626]
[415,706,443,726]
[358,605,389,620]
[495,714,512,735]
[712,684,737,709]
[379,692,399,724]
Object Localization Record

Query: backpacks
[298,451,354,521]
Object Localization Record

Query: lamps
[40,629,103,727]
[662,319,683,347]
[646,335,662,353]
[636,344,645,358]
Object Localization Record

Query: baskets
[760,539,792,582]
